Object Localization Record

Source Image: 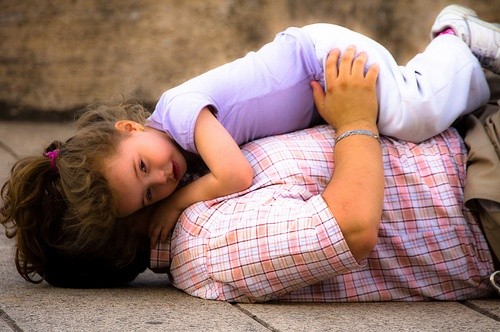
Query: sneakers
[428,4,500,74]
[481,67,500,99]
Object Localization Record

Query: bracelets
[333,130,382,145]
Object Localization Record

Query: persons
[16,45,500,305]
[1,4,499,249]
